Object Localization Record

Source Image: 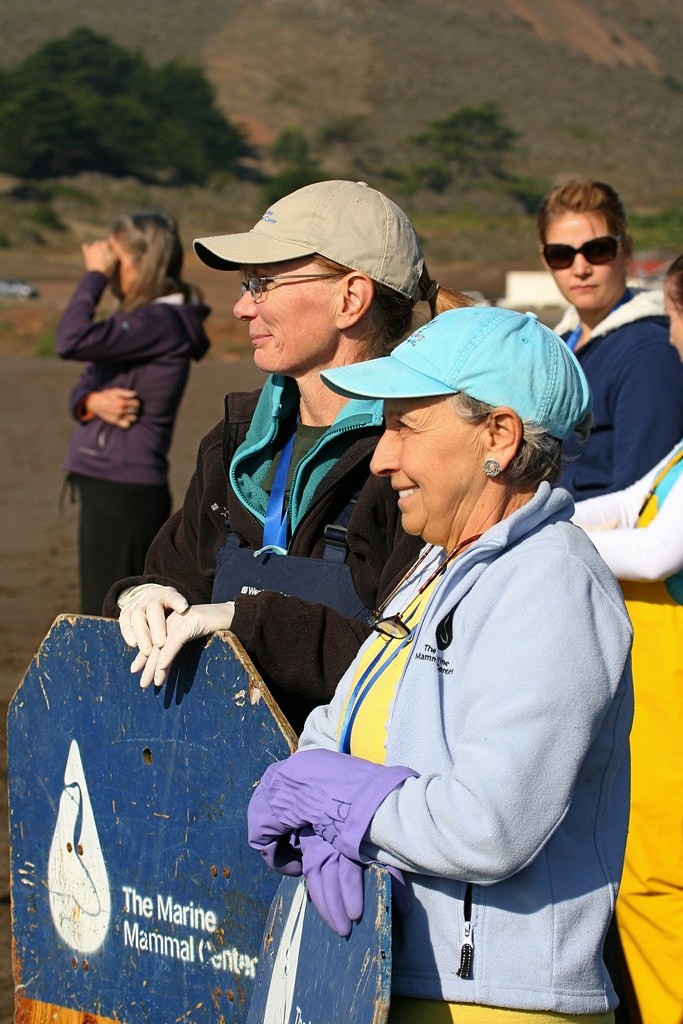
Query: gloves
[247,749,421,937]
[118,583,233,689]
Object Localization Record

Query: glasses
[239,274,348,300]
[537,233,626,271]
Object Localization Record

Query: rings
[128,406,135,414]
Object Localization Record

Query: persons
[534,173,683,504]
[57,214,211,616]
[102,180,479,737]
[248,308,635,1024]
[571,256,683,1024]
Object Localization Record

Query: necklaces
[337,566,446,755]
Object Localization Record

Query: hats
[320,306,590,441]
[192,180,440,314]
